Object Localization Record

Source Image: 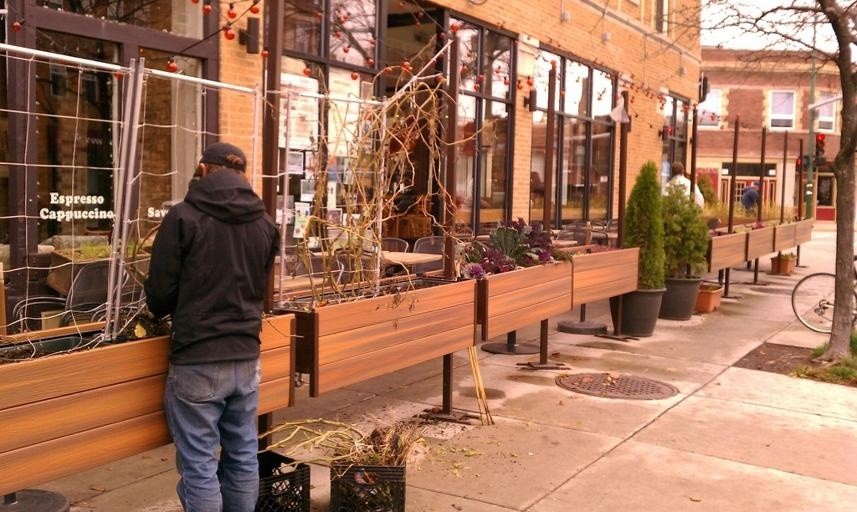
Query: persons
[145,142,282,511]
[659,161,706,209]
[740,188,759,211]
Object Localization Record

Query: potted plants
[610,160,666,338]
[658,181,709,321]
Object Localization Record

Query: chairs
[335,217,618,277]
[292,236,466,292]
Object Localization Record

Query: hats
[193,142,247,177]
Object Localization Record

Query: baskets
[255,450,311,512]
[329,444,406,512]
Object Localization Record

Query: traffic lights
[815,134,824,156]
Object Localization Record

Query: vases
[694,282,723,313]
[770,257,797,274]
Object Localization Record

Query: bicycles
[790,255,857,334]
